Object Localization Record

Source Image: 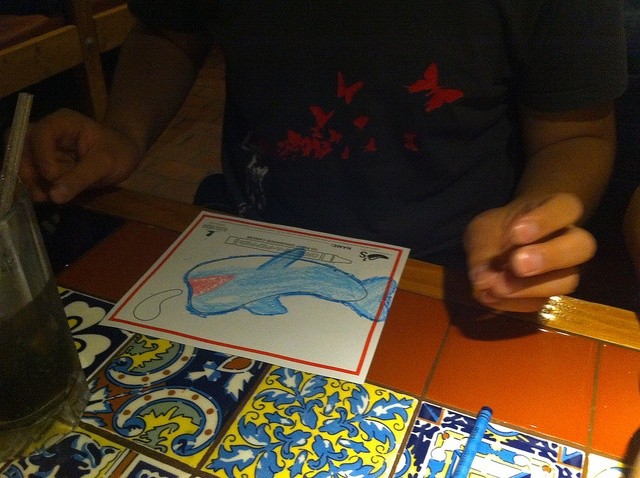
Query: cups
[0,175,90,461]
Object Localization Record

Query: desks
[0,185,640,478]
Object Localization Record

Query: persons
[18,0,631,314]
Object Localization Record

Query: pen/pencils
[1,91,34,217]
[445,405,493,478]
